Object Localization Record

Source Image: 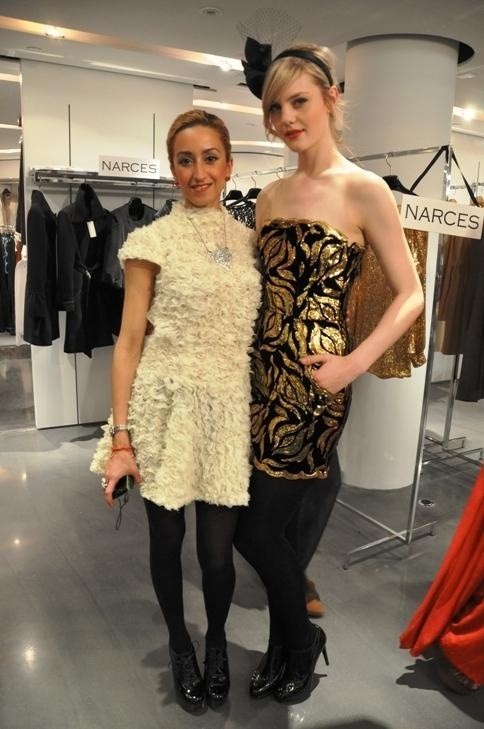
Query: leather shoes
[168,635,208,715]
[202,624,230,710]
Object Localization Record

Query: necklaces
[187,209,233,263]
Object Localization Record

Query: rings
[99,477,107,490]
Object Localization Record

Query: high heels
[247,623,330,703]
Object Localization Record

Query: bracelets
[112,446,132,452]
[110,423,129,435]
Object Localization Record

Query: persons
[231,34,425,707]
[88,108,267,717]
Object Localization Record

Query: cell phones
[111,474,130,499]
